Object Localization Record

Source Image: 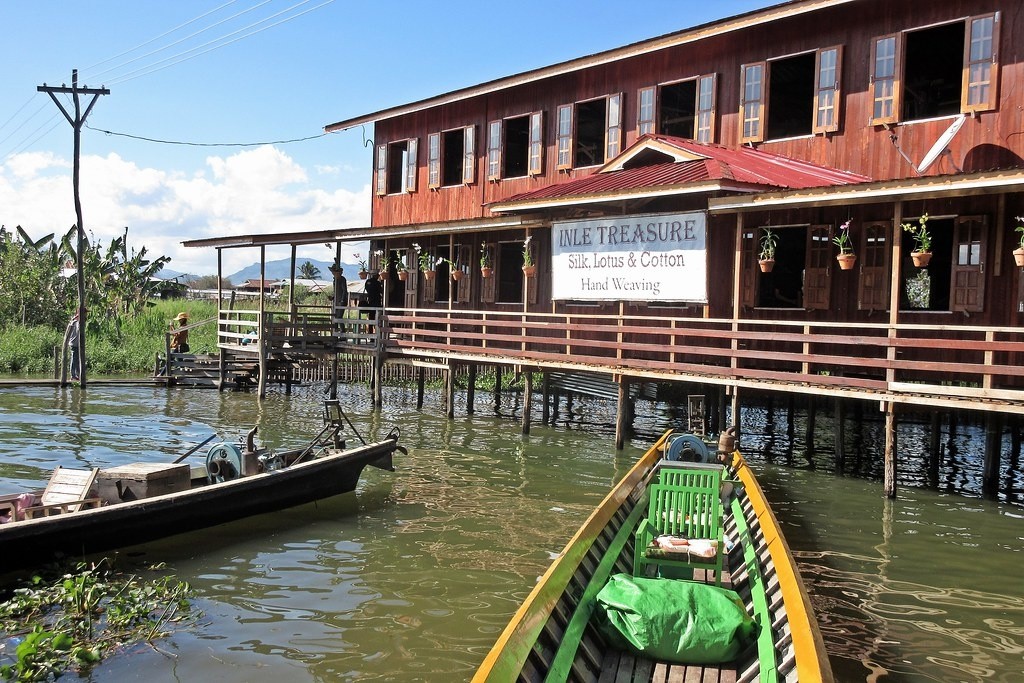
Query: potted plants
[831,220,858,271]
[480,241,492,278]
[353,253,368,279]
[379,257,392,279]
[393,251,410,281]
[521,247,536,277]
[412,243,435,280]
[435,257,463,280]
[757,229,780,273]
[899,213,932,268]
[1012,216,1024,267]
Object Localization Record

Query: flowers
[295,260,329,299]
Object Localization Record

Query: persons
[68,307,92,381]
[326,264,350,344]
[364,273,383,343]
[152,312,190,380]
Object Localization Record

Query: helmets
[370,269,380,276]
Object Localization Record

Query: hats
[173,313,192,321]
[329,264,343,272]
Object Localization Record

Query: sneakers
[154,374,163,381]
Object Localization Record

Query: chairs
[657,468,722,532]
[632,482,723,588]
[0,464,101,523]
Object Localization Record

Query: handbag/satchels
[358,301,370,313]
[178,344,189,352]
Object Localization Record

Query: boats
[0,358,415,569]
[469,428,836,683]
